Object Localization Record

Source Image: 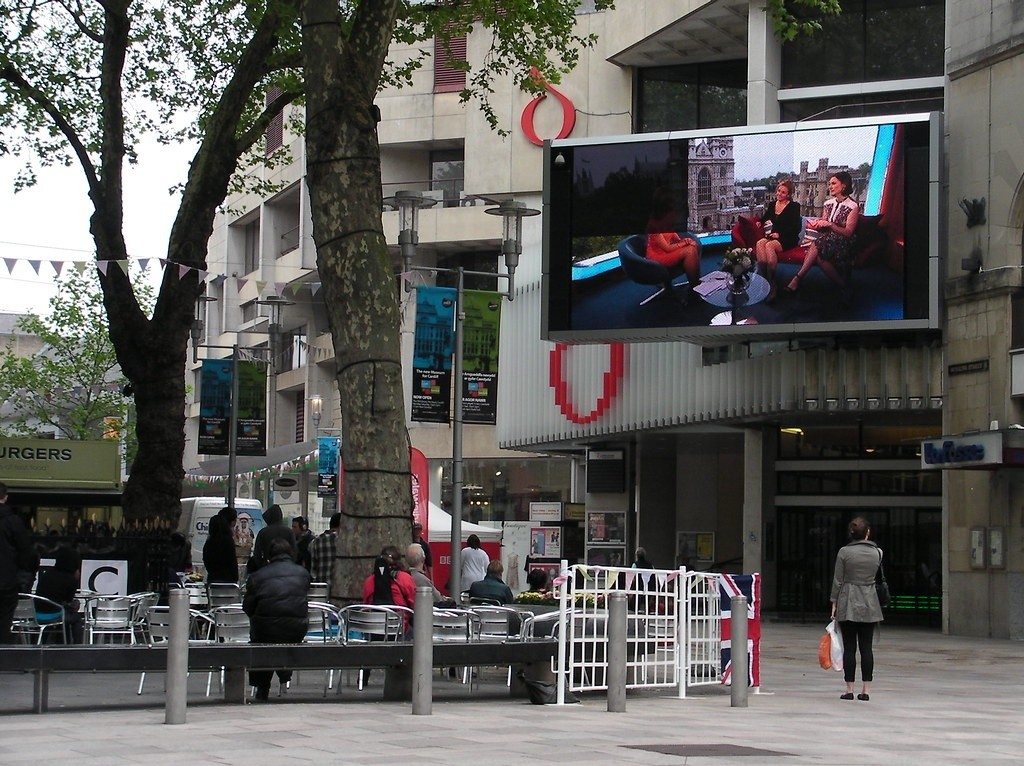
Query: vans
[180,497,266,579]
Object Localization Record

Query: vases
[723,268,750,294]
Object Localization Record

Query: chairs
[61,586,99,644]
[469,595,503,606]
[462,604,537,690]
[136,598,217,697]
[121,591,164,644]
[109,592,156,643]
[506,602,589,689]
[186,582,208,639]
[166,580,183,640]
[207,603,255,697]
[433,608,483,693]
[302,582,413,690]
[82,596,138,645]
[11,590,67,645]
[206,581,241,611]
[616,229,704,305]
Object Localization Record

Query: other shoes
[450,671,478,678]
[256,686,269,700]
[858,694,869,700]
[841,693,853,700]
[786,274,801,294]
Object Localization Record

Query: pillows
[856,210,885,246]
[802,215,823,247]
[798,213,808,246]
[737,211,764,240]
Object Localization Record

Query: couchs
[727,208,891,298]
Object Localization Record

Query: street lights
[383,189,545,614]
[192,293,297,511]
[299,394,324,530]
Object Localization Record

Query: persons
[203,507,238,587]
[357,524,456,686]
[168,532,194,572]
[529,569,547,591]
[0,482,29,644]
[34,546,81,644]
[830,516,885,702]
[631,547,655,613]
[532,540,538,554]
[253,505,297,562]
[469,560,514,603]
[551,532,559,543]
[646,233,700,304]
[233,513,254,555]
[292,513,341,583]
[786,171,859,295]
[242,538,311,699]
[756,181,802,302]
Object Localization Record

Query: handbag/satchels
[819,632,832,670]
[876,548,890,605]
[826,618,844,671]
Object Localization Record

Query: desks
[74,591,138,645]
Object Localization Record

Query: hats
[413,523,422,530]
[236,513,252,529]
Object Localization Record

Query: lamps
[960,248,982,274]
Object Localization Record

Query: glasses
[240,521,247,523]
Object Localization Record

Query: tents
[425,502,503,596]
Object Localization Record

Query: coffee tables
[696,269,772,328]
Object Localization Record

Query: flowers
[188,566,206,582]
[721,242,757,272]
